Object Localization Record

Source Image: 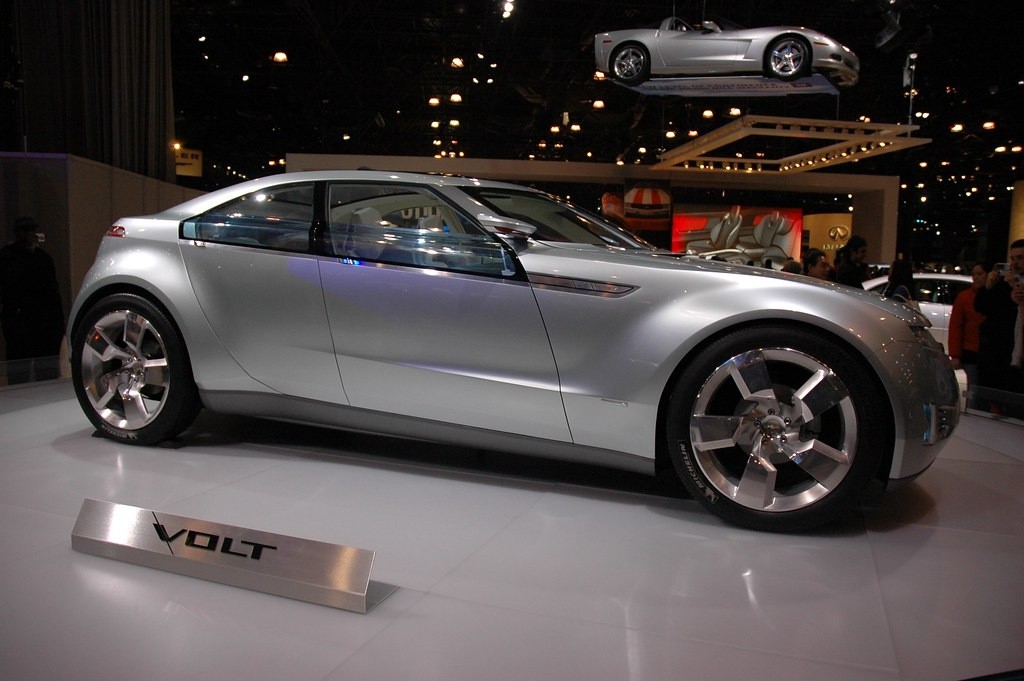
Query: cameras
[36,233,45,242]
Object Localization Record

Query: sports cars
[625,180,671,220]
[593,14,861,90]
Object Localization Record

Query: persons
[711,234,1024,421]
[0,216,68,385]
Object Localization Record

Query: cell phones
[995,263,1009,275]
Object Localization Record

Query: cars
[858,272,974,356]
[65,171,966,534]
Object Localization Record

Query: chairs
[420,214,445,265]
[354,208,386,259]
